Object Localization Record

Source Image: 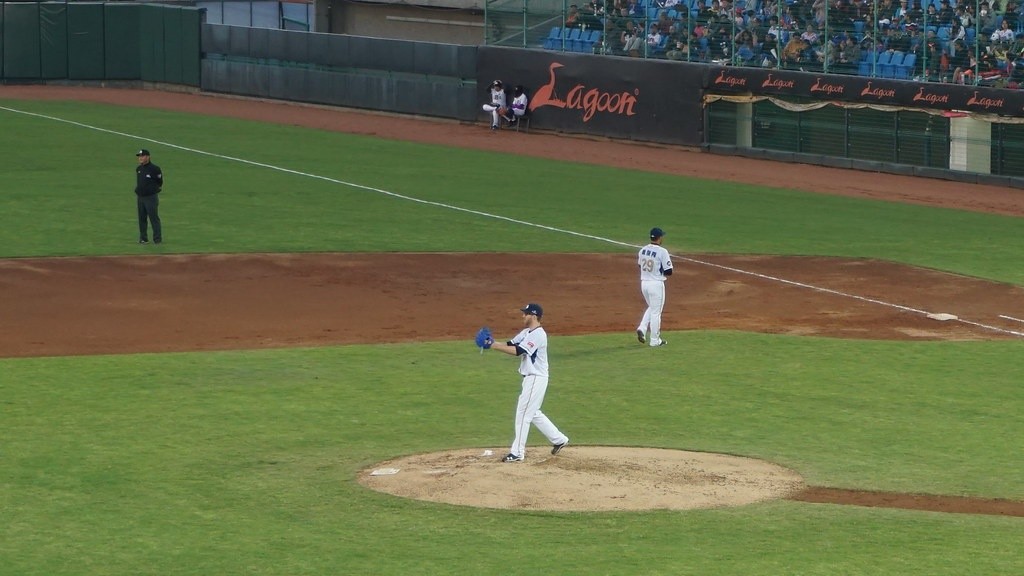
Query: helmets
[493,80,503,86]
[512,85,523,98]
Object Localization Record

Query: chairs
[541,0,1023,81]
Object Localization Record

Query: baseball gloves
[475,326,495,354]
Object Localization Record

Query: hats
[735,7,742,14]
[990,33,999,41]
[519,303,544,319]
[136,150,151,156]
[650,228,666,238]
[940,0,951,5]
[892,18,899,22]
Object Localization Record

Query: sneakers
[551,438,570,454]
[502,453,525,463]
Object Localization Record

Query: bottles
[943,77,947,84]
[762,57,769,68]
[925,70,929,83]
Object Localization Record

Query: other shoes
[659,339,672,347]
[490,125,498,130]
[507,121,517,127]
[137,240,150,245]
[637,329,646,344]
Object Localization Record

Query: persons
[504,82,528,127]
[567,0,1024,91]
[135,149,164,245]
[636,227,673,347]
[475,303,570,462]
[483,79,507,130]
